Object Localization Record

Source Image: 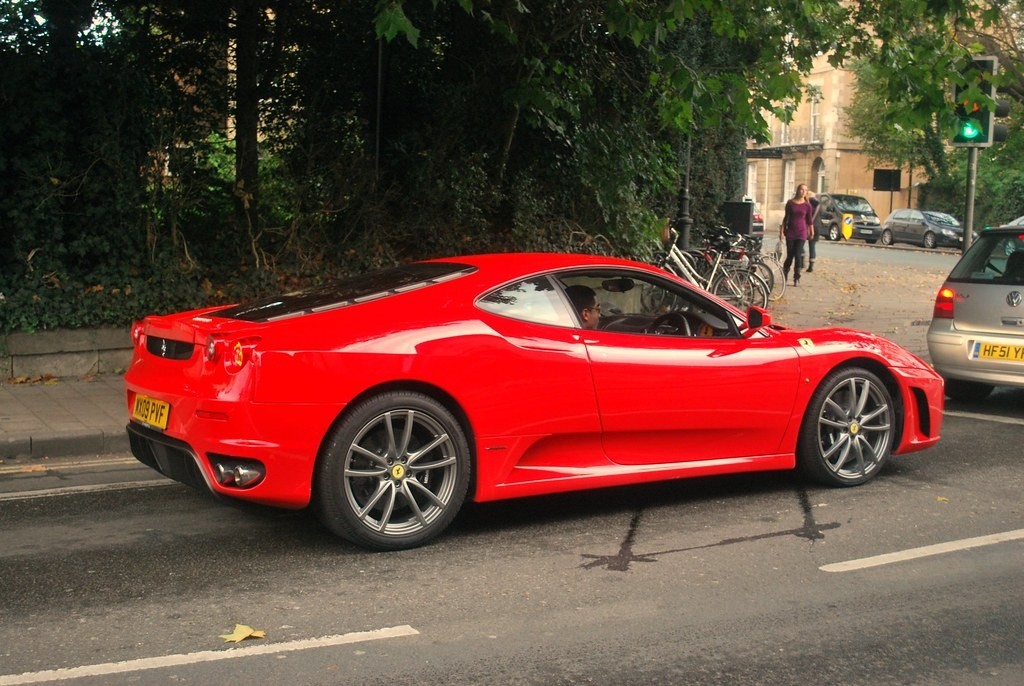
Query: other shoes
[794,279,799,287]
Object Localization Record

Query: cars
[816,193,882,244]
[752,203,764,251]
[927,215,1024,401]
[881,209,978,249]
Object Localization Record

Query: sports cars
[124,254,945,551]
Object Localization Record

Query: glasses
[585,306,601,314]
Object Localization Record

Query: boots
[806,258,815,272]
[801,253,806,267]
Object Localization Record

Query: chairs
[1004,249,1024,279]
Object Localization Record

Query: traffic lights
[948,56,997,146]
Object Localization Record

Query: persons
[801,191,820,272]
[779,184,814,287]
[564,285,600,330]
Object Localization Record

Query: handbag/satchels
[774,225,784,262]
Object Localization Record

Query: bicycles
[660,226,786,315]
